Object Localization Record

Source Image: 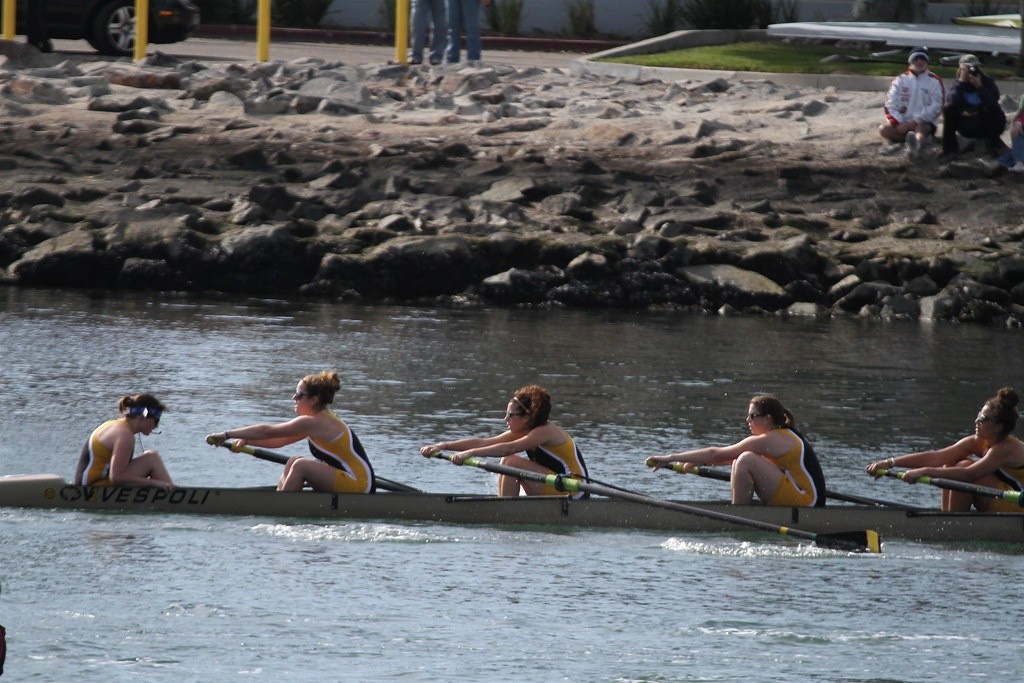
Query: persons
[867,387,1024,512]
[645,396,826,508]
[420,384,590,499]
[206,370,376,494]
[973,93,1024,176]
[878,46,945,163]
[75,393,173,490]
[934,54,1013,157]
[447,0,495,65]
[408,0,447,65]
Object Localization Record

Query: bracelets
[890,456,895,467]
[441,441,448,449]
[225,429,228,439]
[886,458,891,468]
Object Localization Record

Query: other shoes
[935,143,959,159]
[905,130,917,163]
[1007,165,1024,173]
[976,159,993,178]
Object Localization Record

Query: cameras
[965,64,979,76]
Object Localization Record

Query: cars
[0,0,201,56]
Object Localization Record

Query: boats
[1,475,1024,543]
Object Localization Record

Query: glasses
[506,412,523,418]
[976,411,996,424]
[747,413,766,420]
[295,391,310,401]
[149,415,160,426]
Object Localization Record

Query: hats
[959,54,982,67]
[907,46,930,62]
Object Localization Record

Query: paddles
[207,437,422,493]
[864,465,1024,508]
[646,458,906,507]
[420,448,881,554]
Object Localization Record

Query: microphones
[151,431,162,434]
